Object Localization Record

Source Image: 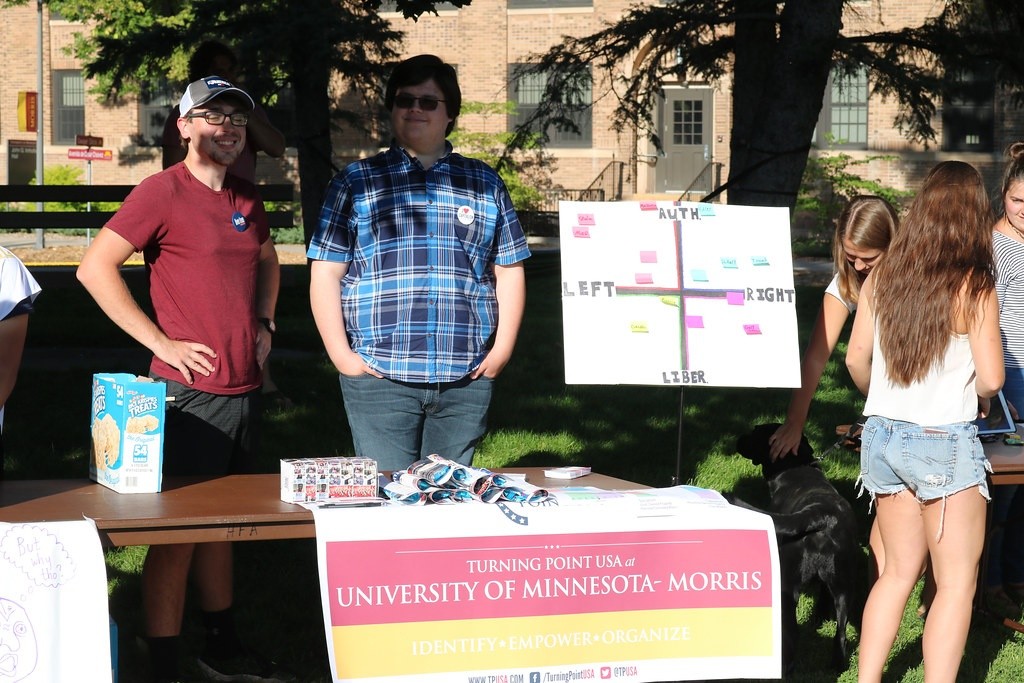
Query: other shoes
[1003,583,1024,604]
[976,585,1021,622]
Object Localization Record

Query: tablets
[970,390,1017,435]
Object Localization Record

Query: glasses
[188,110,250,127]
[393,94,446,111]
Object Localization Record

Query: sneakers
[197,648,297,683]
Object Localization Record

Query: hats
[179,75,255,117]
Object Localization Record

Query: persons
[1,242,41,413]
[303,55,531,465]
[771,139,1024,683]
[844,161,1007,683]
[75,72,281,683]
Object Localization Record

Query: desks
[0,467,765,683]
[836,423,1024,634]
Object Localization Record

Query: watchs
[259,317,276,332]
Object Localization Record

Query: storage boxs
[88,372,165,494]
[280,457,378,503]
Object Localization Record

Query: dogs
[721,422,865,681]
